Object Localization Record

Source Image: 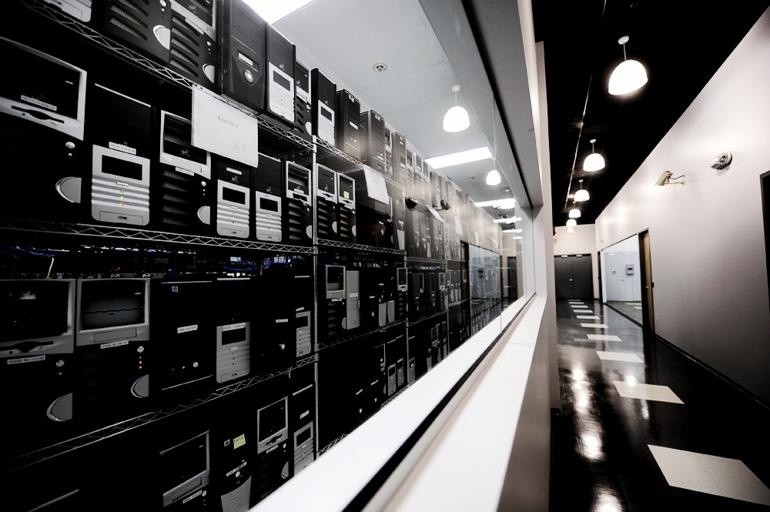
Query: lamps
[566,218,577,229]
[569,201,581,219]
[608,35,649,96]
[574,179,590,202]
[583,139,605,173]
[442,84,471,133]
[485,157,501,186]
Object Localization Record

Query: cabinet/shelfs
[0,1,502,512]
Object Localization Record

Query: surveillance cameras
[654,170,673,186]
